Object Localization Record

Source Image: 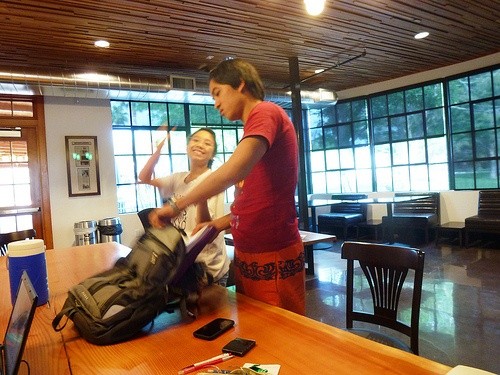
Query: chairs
[0,228,37,257]
[341,241,426,356]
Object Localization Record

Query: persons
[147,59,307,319]
[138,128,232,286]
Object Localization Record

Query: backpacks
[51,218,214,345]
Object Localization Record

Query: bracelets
[167,198,181,215]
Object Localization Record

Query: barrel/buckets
[5,238,50,309]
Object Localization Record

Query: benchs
[465,190,500,247]
[382,192,440,244]
[318,195,367,241]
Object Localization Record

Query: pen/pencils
[179,353,233,374]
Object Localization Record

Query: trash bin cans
[99,217,122,244]
[74,220,98,246]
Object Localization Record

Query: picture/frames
[65,135,101,197]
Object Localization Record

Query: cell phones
[193,317,235,340]
[221,337,256,358]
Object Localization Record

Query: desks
[295,199,352,249]
[224,229,336,274]
[0,242,454,375]
[357,220,382,240]
[348,196,432,243]
[441,222,465,246]
